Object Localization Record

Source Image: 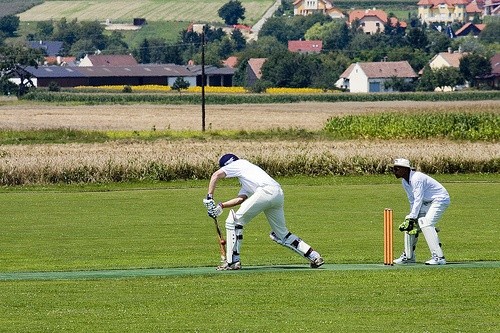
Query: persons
[203,154,325,271]
[387,159,450,265]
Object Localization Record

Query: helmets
[219,154,239,168]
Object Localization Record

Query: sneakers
[310,257,324,268]
[215,260,242,270]
[425,256,446,265]
[393,253,416,265]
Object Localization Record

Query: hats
[390,159,411,167]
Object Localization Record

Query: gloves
[398,219,414,232]
[408,223,421,234]
[208,202,224,218]
[202,193,215,209]
